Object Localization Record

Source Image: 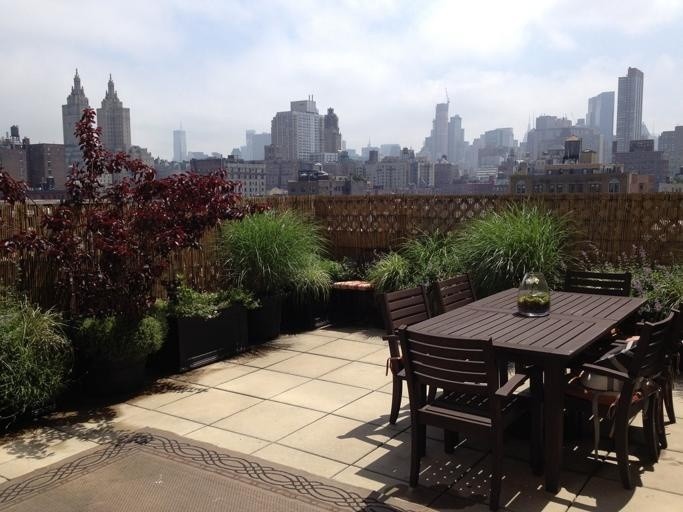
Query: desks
[408,287,648,494]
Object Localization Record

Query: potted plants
[211,208,333,347]
[150,272,264,377]
[65,314,170,397]
[282,268,332,333]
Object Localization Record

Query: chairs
[555,307,680,490]
[555,270,632,380]
[393,321,537,512]
[382,273,509,425]
